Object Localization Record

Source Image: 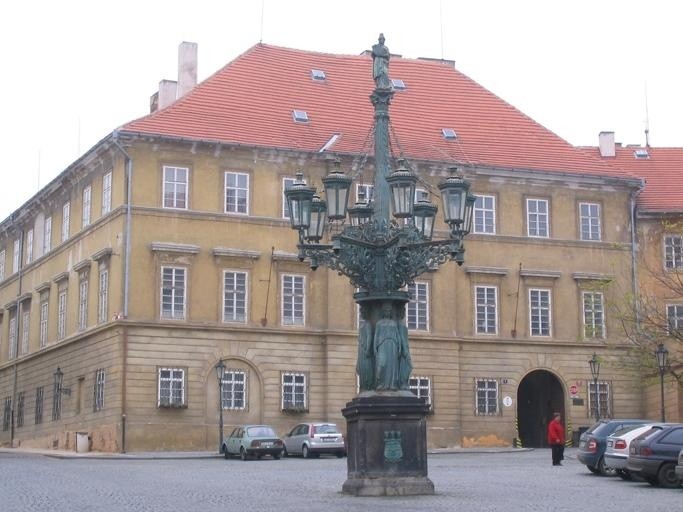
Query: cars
[627,423,683,489]
[220,423,285,461]
[279,420,347,459]
[575,417,657,477]
[605,421,675,483]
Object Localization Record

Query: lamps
[52,366,71,395]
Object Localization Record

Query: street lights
[213,355,228,455]
[654,341,669,423]
[587,352,603,422]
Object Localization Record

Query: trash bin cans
[572,427,589,448]
[76,432,88,453]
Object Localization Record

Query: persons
[547,411,565,467]
